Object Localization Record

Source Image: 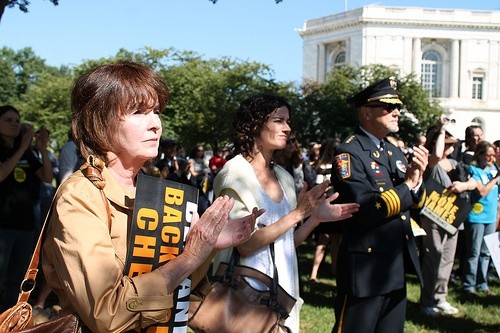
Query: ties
[379,139,389,163]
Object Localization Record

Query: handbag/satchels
[0,302,88,333]
[187,222,297,333]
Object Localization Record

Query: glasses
[286,137,296,145]
[363,104,402,113]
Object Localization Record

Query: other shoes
[436,299,458,315]
[420,302,441,317]
[463,287,482,299]
[478,288,497,296]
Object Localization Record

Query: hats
[160,139,177,148]
[345,74,404,109]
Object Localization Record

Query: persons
[386,113,500,333]
[213,93,360,333]
[42,61,265,333]
[59,128,78,183]
[274,129,339,290]
[0,105,59,309]
[156,139,233,218]
[330,75,429,333]
